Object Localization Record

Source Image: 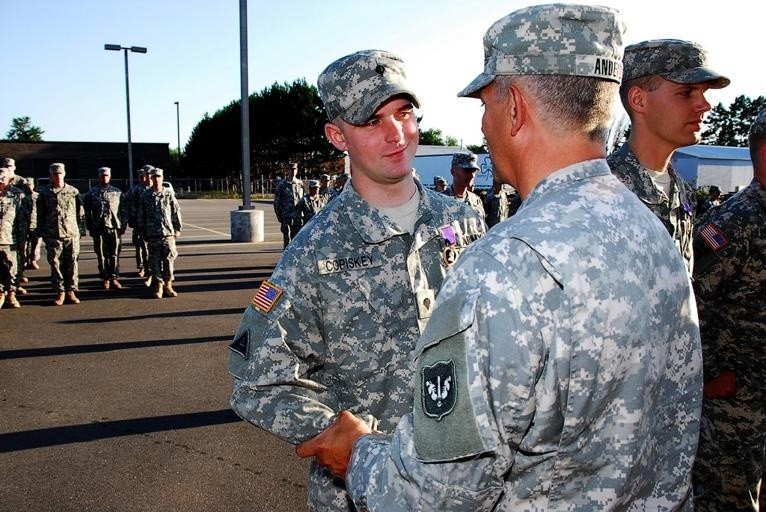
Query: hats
[623,38,731,92]
[287,162,298,170]
[143,164,155,174]
[49,163,66,175]
[150,167,163,177]
[139,168,144,174]
[321,173,330,182]
[449,152,480,172]
[97,167,112,176]
[4,157,15,166]
[309,179,321,188]
[455,3,627,97]
[316,49,420,127]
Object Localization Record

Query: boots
[153,282,164,298]
[113,279,122,289]
[18,287,27,295]
[165,281,177,296]
[138,269,144,278]
[20,277,29,284]
[54,292,65,305]
[9,292,21,308]
[29,260,40,270]
[67,291,81,304]
[104,280,110,289]
[0,293,5,308]
[144,275,152,287]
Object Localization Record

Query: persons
[693,101,765,511]
[83,165,129,290]
[0,155,42,310]
[483,177,512,227]
[225,43,491,512]
[320,172,335,200]
[128,164,183,299]
[430,173,450,195]
[35,161,85,305]
[290,0,706,512]
[701,184,721,210]
[439,151,498,226]
[606,36,732,281]
[293,179,327,235]
[272,161,304,251]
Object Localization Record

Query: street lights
[105,44,147,189]
